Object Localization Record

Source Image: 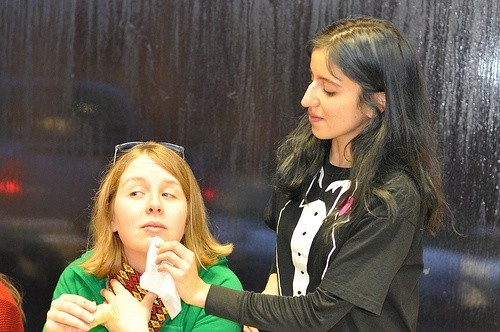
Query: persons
[18,240,60,332]
[155,15,447,332]
[42,141,245,332]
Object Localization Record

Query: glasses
[113,142,185,165]
